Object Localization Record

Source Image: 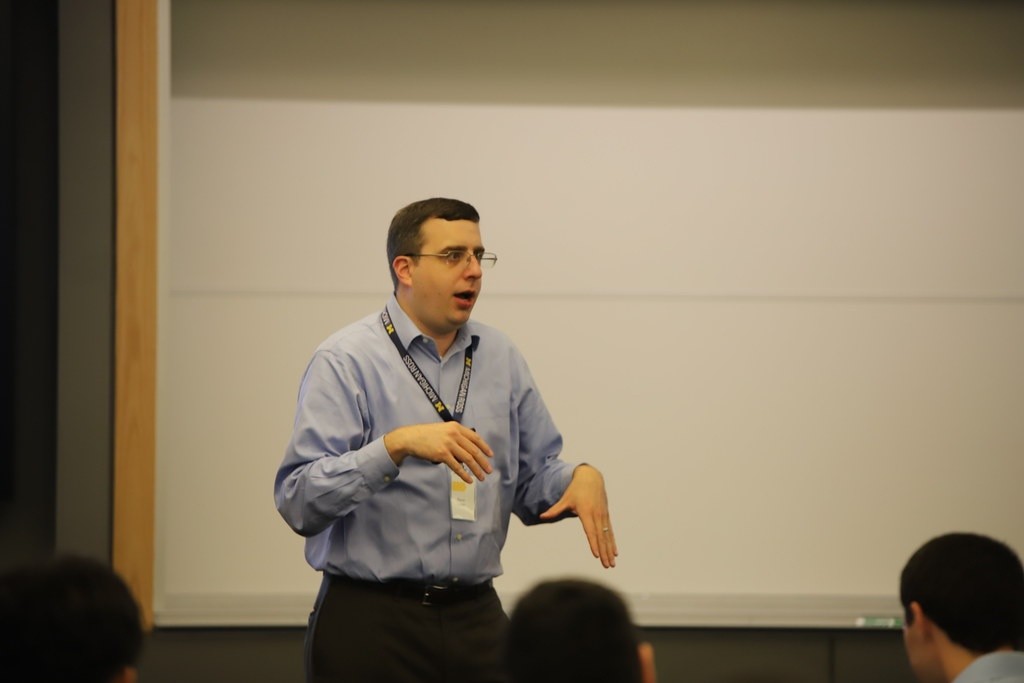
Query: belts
[324,571,492,606]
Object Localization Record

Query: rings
[603,528,608,532]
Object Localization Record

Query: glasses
[403,250,497,272]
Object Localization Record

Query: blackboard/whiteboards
[108,0,1023,640]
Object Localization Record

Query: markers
[431,428,477,465]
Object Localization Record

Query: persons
[0,557,144,683]
[273,196,619,683]
[899,531,1024,683]
[501,577,656,683]
